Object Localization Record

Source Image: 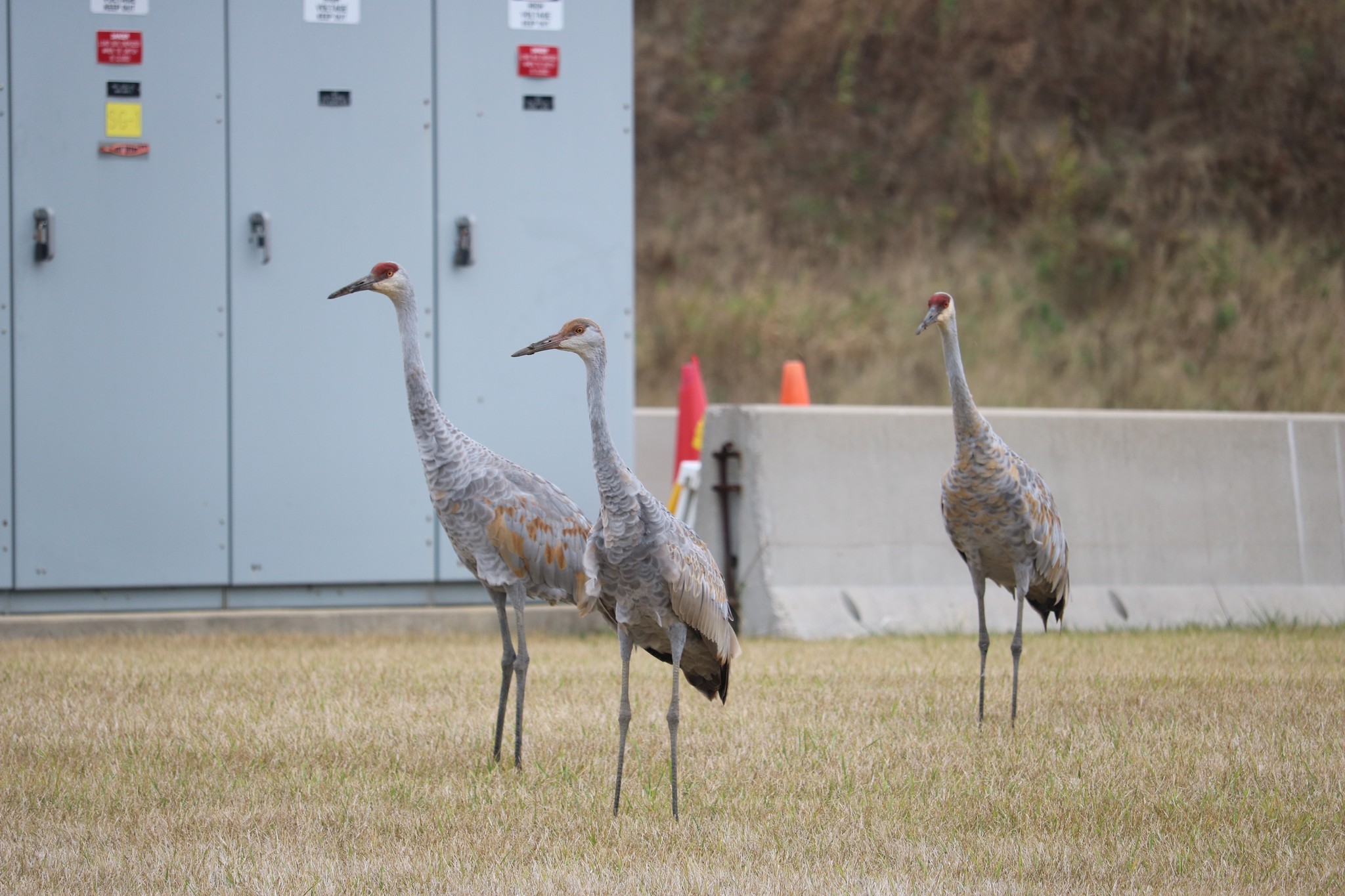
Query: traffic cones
[670,350,711,487]
[775,359,811,405]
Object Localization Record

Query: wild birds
[507,318,740,824]
[327,255,592,768]
[917,288,1073,738]
